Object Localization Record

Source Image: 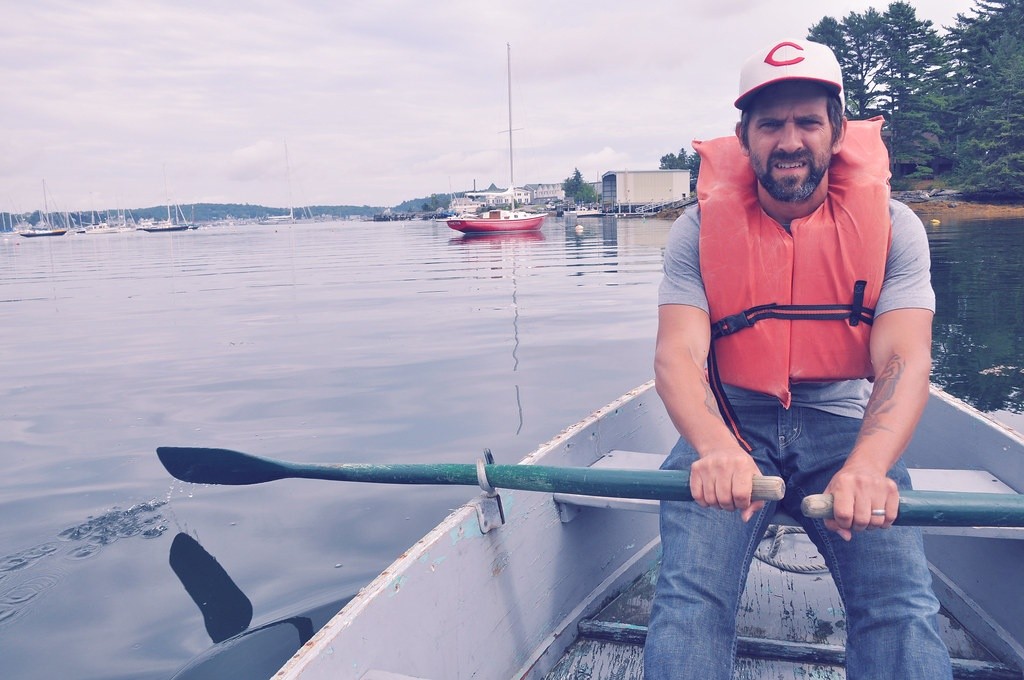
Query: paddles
[796,491,1024,529]
[152,441,791,503]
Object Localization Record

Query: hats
[733,39,845,116]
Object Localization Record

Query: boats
[562,199,606,217]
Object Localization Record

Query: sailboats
[0,163,202,239]
[435,41,550,233]
[257,139,316,225]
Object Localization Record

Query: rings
[870,509,888,516]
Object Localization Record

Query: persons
[644,37,954,680]
